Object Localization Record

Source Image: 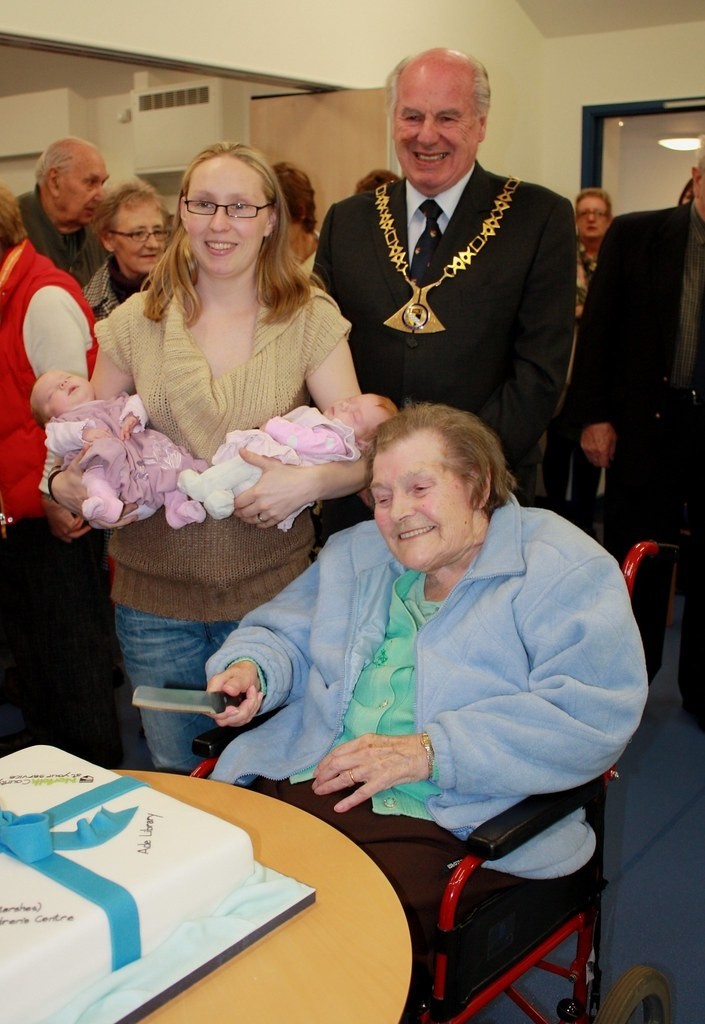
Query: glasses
[111,229,171,242]
[184,195,272,218]
[579,209,607,218]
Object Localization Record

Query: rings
[349,769,357,783]
[257,513,267,522]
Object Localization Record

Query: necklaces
[376,173,522,334]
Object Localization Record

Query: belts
[664,389,704,406]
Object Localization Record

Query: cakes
[0,744,255,1024]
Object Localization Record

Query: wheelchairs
[187,542,686,1024]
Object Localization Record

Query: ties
[410,200,443,285]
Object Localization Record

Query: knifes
[132,685,246,716]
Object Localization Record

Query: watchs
[420,732,434,777]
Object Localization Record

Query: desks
[95,768,412,1024]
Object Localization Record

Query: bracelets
[48,470,62,504]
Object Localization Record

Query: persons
[542,152,705,733]
[0,137,403,771]
[43,142,375,786]
[177,394,398,533]
[206,405,649,965]
[30,370,209,530]
[307,49,575,550]
